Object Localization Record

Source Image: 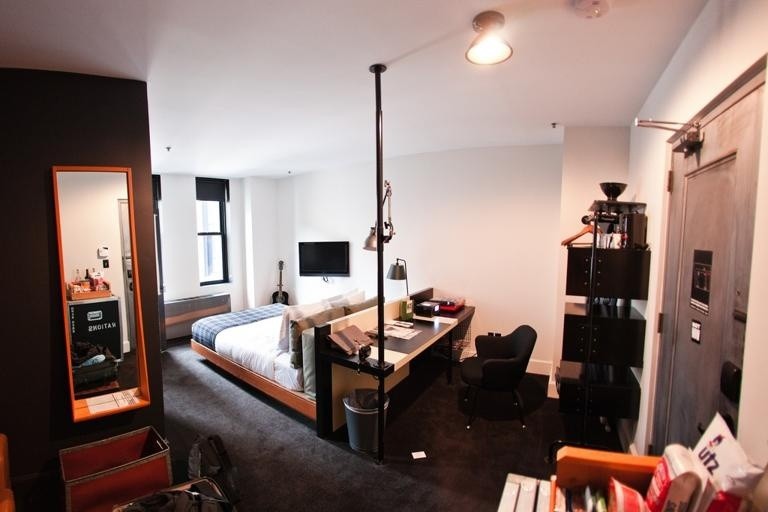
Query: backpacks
[187,434,240,504]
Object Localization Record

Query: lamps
[464,11,514,65]
[363,186,394,252]
[386,258,413,321]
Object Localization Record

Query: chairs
[459,324,539,431]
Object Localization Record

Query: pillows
[345,295,386,318]
[288,306,347,370]
[329,291,367,312]
[287,302,332,353]
[323,288,360,307]
[277,301,326,352]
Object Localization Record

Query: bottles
[67,268,108,294]
[187,444,202,480]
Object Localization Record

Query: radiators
[164,293,231,337]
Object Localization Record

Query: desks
[329,298,475,465]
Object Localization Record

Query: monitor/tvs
[299,241,349,277]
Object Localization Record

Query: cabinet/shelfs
[543,197,651,466]
[67,295,124,362]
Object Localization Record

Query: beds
[189,286,434,440]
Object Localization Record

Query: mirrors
[51,166,152,423]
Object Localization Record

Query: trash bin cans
[342,388,390,454]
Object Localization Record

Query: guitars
[272,260,288,304]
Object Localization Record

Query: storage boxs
[58,425,173,511]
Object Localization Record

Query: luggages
[111,477,236,512]
[71,355,120,391]
